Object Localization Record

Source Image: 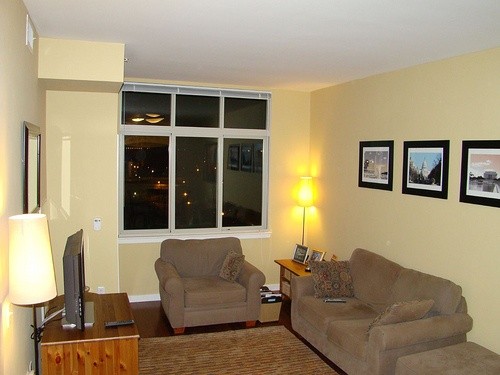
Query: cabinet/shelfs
[40,291,140,375]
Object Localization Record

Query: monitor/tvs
[61,229,95,331]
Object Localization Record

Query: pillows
[367,299,434,333]
[218,251,245,283]
[309,259,355,299]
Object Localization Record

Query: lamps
[292,176,316,246]
[8,215,58,375]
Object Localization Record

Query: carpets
[138,324,338,375]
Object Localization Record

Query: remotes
[104,320,135,327]
[323,299,346,303]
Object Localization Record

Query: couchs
[154,237,266,335]
[290,248,473,374]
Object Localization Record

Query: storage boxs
[259,301,283,323]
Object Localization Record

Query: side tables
[274,258,314,302]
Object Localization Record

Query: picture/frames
[306,249,326,266]
[459,140,500,207]
[241,144,254,172]
[226,144,239,170]
[358,140,394,191]
[253,142,263,173]
[24,123,42,214]
[402,140,449,199]
[292,243,309,264]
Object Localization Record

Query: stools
[394,341,500,375]
